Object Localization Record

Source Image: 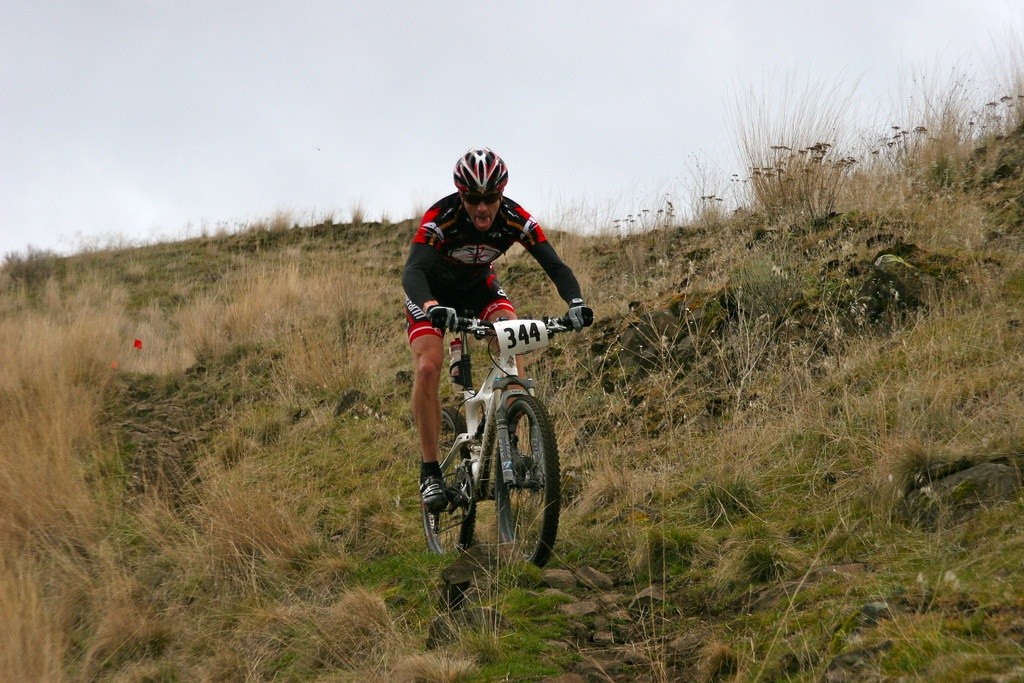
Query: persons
[402,148,594,510]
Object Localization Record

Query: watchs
[569,298,585,308]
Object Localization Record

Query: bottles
[449,337,464,382]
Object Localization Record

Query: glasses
[462,191,500,205]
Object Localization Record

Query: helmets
[453,147,508,192]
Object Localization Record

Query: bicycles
[418,316,575,569]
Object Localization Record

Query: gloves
[426,304,458,333]
[563,298,593,331]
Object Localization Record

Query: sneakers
[419,476,447,509]
[525,470,544,486]
